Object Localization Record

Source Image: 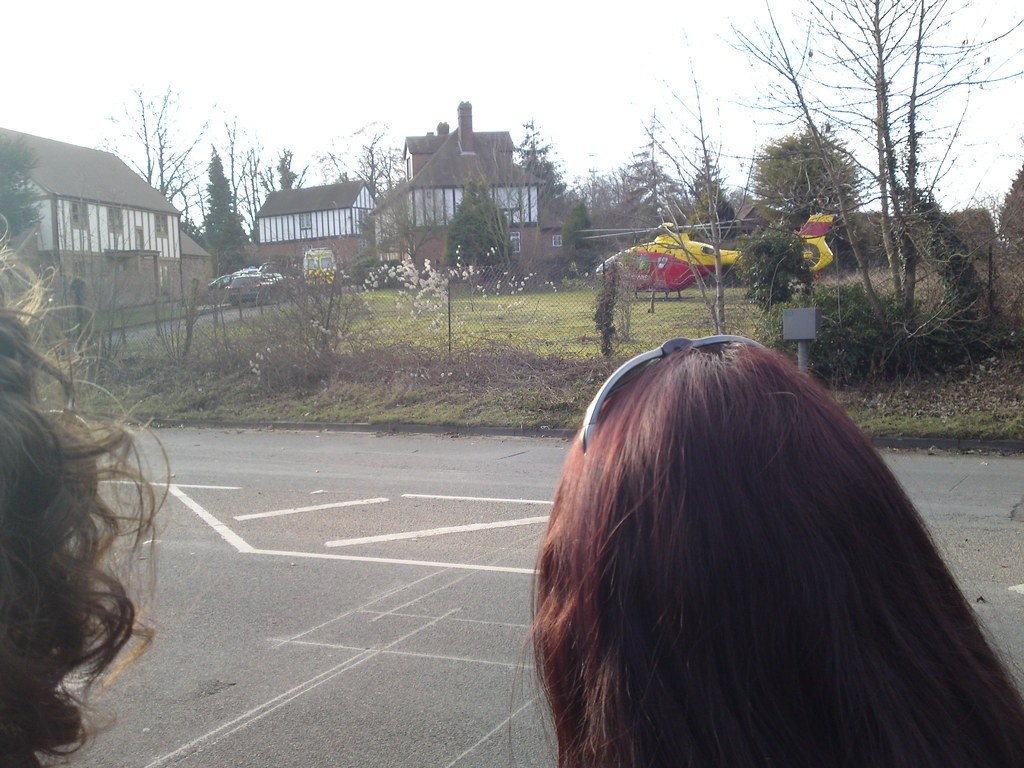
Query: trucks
[302,247,337,289]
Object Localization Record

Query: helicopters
[573,214,838,305]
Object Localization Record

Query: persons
[0,212,175,767]
[500,334,1024,768]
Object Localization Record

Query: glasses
[581,335,767,456]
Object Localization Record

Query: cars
[204,262,285,303]
